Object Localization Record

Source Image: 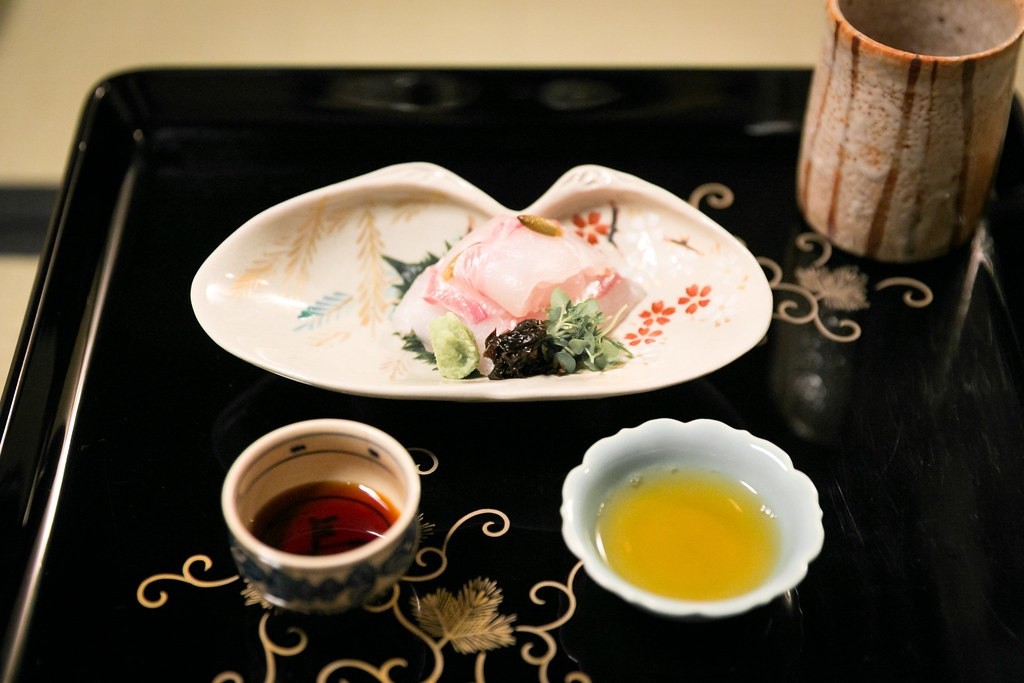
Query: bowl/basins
[220,419,421,615]
[559,416,826,619]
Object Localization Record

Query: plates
[191,163,773,400]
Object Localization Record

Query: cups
[797,0,1024,263]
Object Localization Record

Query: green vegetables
[545,288,636,372]
